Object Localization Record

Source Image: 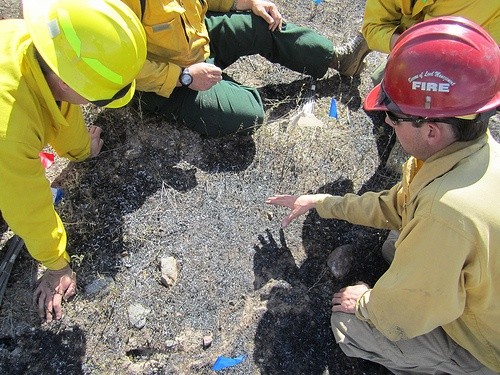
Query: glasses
[385,111,455,127]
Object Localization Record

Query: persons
[120,0,372,139]
[267,17,500,375]
[0,0,147,323]
[362,1,500,87]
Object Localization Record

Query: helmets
[22,1,147,109]
[363,15,500,118]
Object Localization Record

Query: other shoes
[334,30,371,80]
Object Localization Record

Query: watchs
[180,67,194,89]
